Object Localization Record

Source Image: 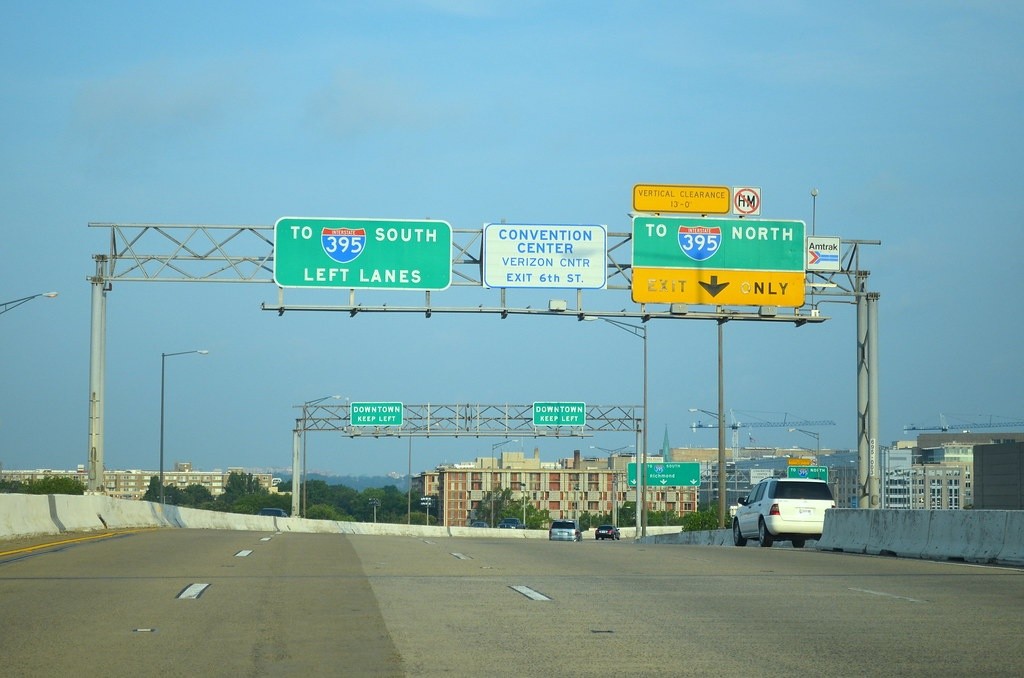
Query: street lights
[491,439,520,528]
[408,421,441,524]
[159,349,210,503]
[419,497,433,525]
[589,444,636,526]
[583,315,650,538]
[302,393,343,519]
[786,428,821,479]
[687,408,727,457]
[368,497,380,523]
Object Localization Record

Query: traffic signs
[626,461,702,488]
[630,215,809,309]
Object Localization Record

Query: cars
[469,521,490,528]
[259,507,289,517]
[595,524,621,541]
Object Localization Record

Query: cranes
[902,410,1024,435]
[691,407,839,461]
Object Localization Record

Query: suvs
[732,476,836,548]
[547,518,583,542]
[497,517,526,530]
